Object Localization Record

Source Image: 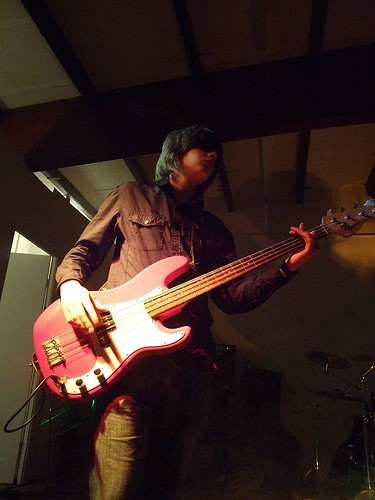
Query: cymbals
[350,355,375,361]
[305,351,352,370]
[306,388,361,402]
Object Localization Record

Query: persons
[56,127,322,500]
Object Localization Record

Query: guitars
[30,197,375,406]
